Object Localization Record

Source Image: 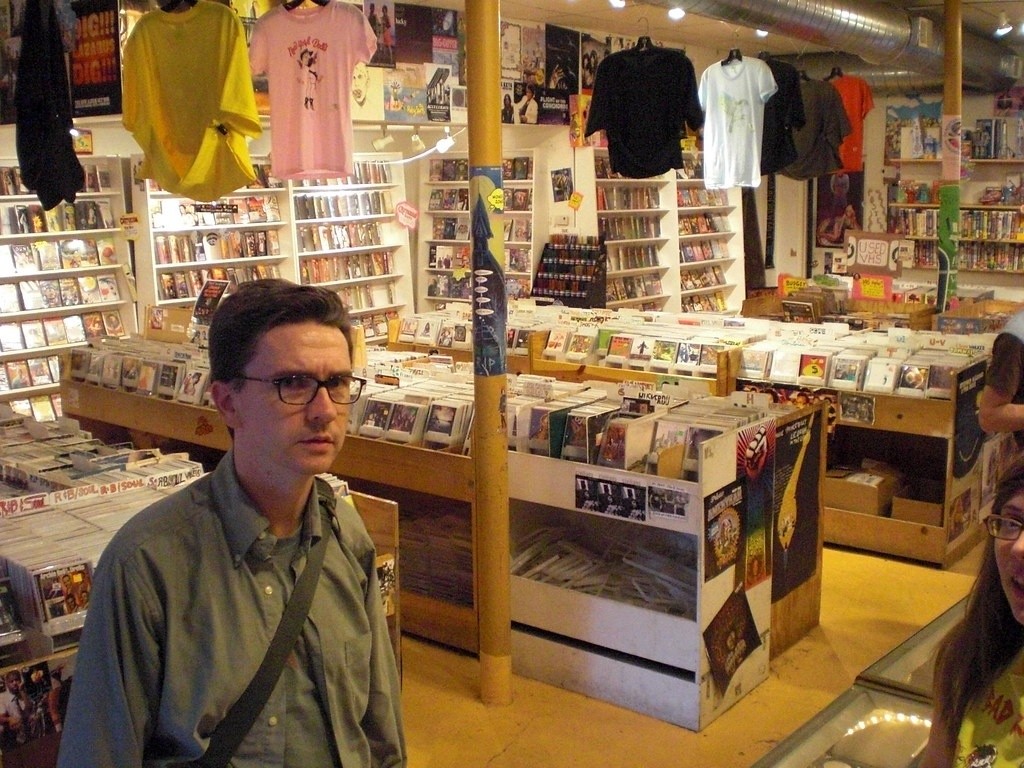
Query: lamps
[995,10,1013,36]
[372,125,455,154]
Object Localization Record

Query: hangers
[621,17,673,58]
[755,36,845,83]
[282,0,331,11]
[160,0,198,13]
[721,32,742,67]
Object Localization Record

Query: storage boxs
[825,456,912,519]
[891,476,946,526]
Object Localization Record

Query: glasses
[220,372,367,405]
[983,514,1024,541]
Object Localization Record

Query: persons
[366,3,394,67]
[0,562,89,755]
[502,94,515,124]
[515,83,539,124]
[918,456,1024,767]
[54,278,408,767]
[978,309,1024,455]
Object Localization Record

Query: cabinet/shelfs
[0,147,1024,768]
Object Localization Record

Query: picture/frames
[191,279,230,328]
